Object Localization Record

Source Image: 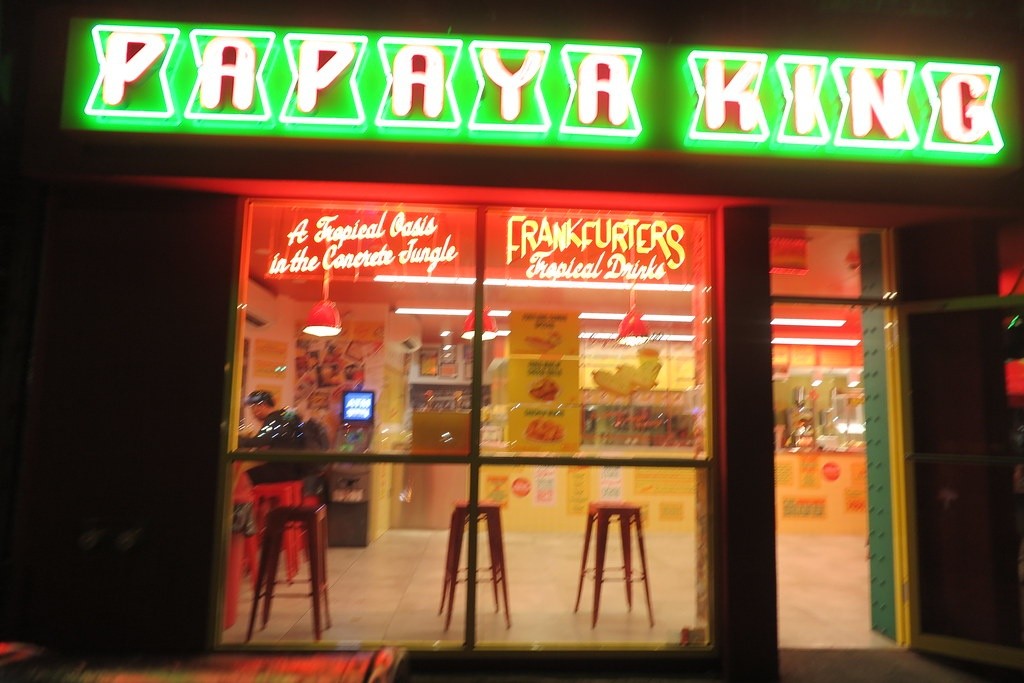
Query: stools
[244,503,332,643]
[437,498,511,628]
[574,502,654,629]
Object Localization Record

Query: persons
[232,389,318,538]
[783,409,815,449]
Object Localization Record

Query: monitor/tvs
[413,410,471,456]
[341,390,374,424]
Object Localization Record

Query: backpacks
[271,412,330,473]
